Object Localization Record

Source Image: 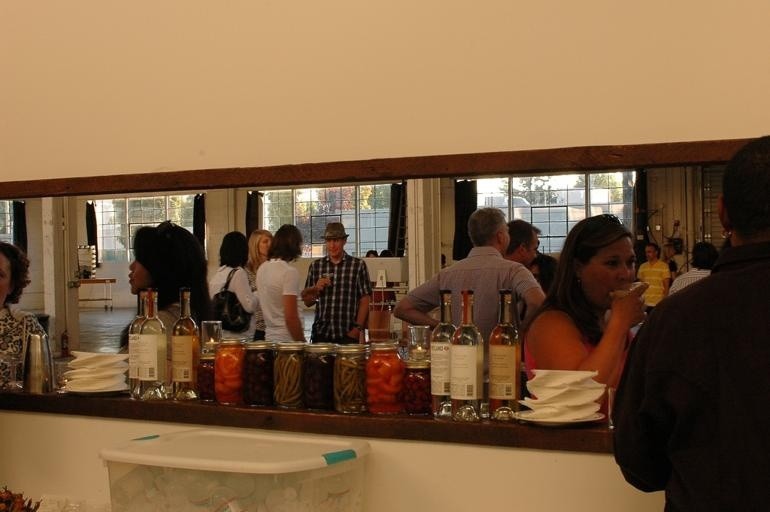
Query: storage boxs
[92,427,372,512]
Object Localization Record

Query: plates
[59,385,131,393]
[67,360,128,372]
[510,410,606,424]
[62,367,125,379]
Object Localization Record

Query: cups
[619,281,645,297]
[323,273,334,285]
[202,321,223,357]
[408,325,431,364]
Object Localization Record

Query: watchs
[352,323,364,332]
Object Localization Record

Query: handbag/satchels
[212,267,255,333]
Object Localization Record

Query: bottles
[430,290,458,420]
[216,336,245,408]
[274,346,303,411]
[244,342,274,408]
[138,287,167,397]
[450,290,484,423]
[128,290,149,398]
[302,343,335,413]
[364,343,406,416]
[404,360,431,416]
[489,289,523,422]
[171,289,199,402]
[198,354,216,404]
[333,344,369,415]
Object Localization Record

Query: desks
[77,278,116,312]
[371,287,409,312]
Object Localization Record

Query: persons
[525,254,558,294]
[525,214,650,423]
[503,220,541,266]
[256,223,307,342]
[119,222,214,355]
[301,224,371,343]
[208,231,260,342]
[669,242,718,299]
[612,135,770,512]
[637,243,669,315]
[243,229,274,342]
[0,240,48,390]
[662,244,678,287]
[393,208,546,393]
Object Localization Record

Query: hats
[321,222,349,240]
[132,221,205,302]
